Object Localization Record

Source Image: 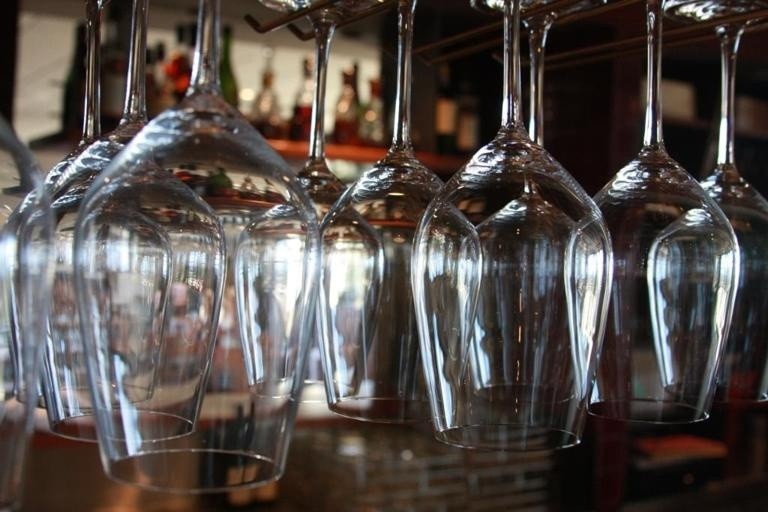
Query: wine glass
[0,1,614,510]
[562,1,741,433]
[680,4,768,406]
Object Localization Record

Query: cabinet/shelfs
[0,121,466,230]
